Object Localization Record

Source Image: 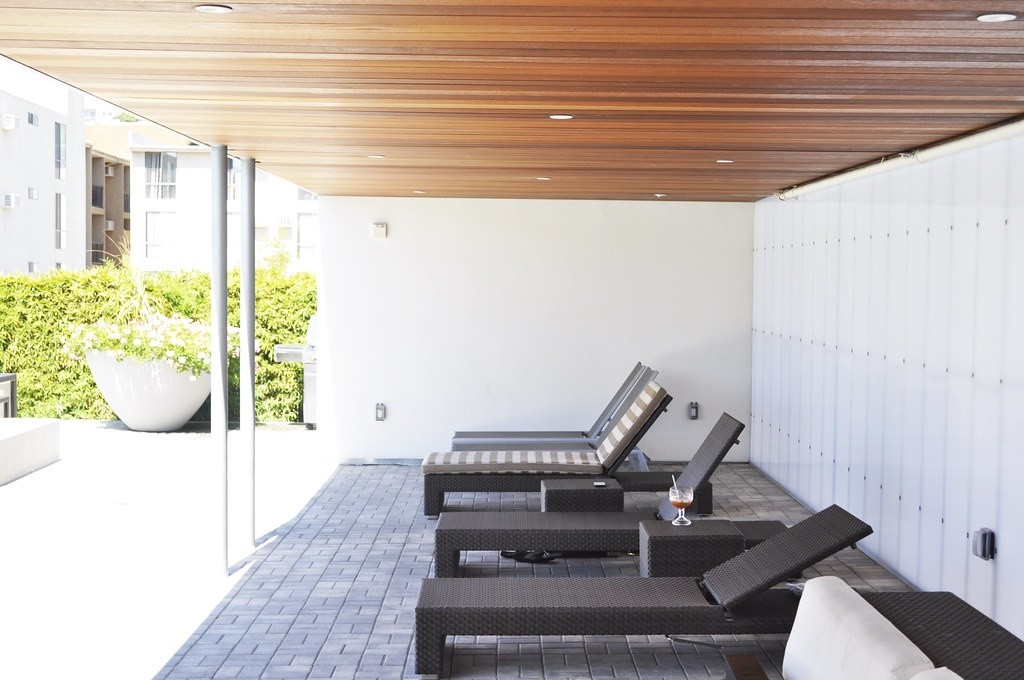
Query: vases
[85,350,212,432]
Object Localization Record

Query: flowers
[62,231,241,380]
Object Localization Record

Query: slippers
[514,550,551,564]
[500,550,532,558]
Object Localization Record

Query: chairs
[450,367,659,461]
[452,361,645,442]
[422,381,713,518]
[434,413,791,578]
[414,502,873,674]
[861,591,1024,680]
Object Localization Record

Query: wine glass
[669,486,693,526]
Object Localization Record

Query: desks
[540,478,626,557]
[638,519,746,578]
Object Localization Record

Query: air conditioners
[105,219,114,231]
[3,194,20,208]
[3,114,20,130]
[105,165,114,177]
